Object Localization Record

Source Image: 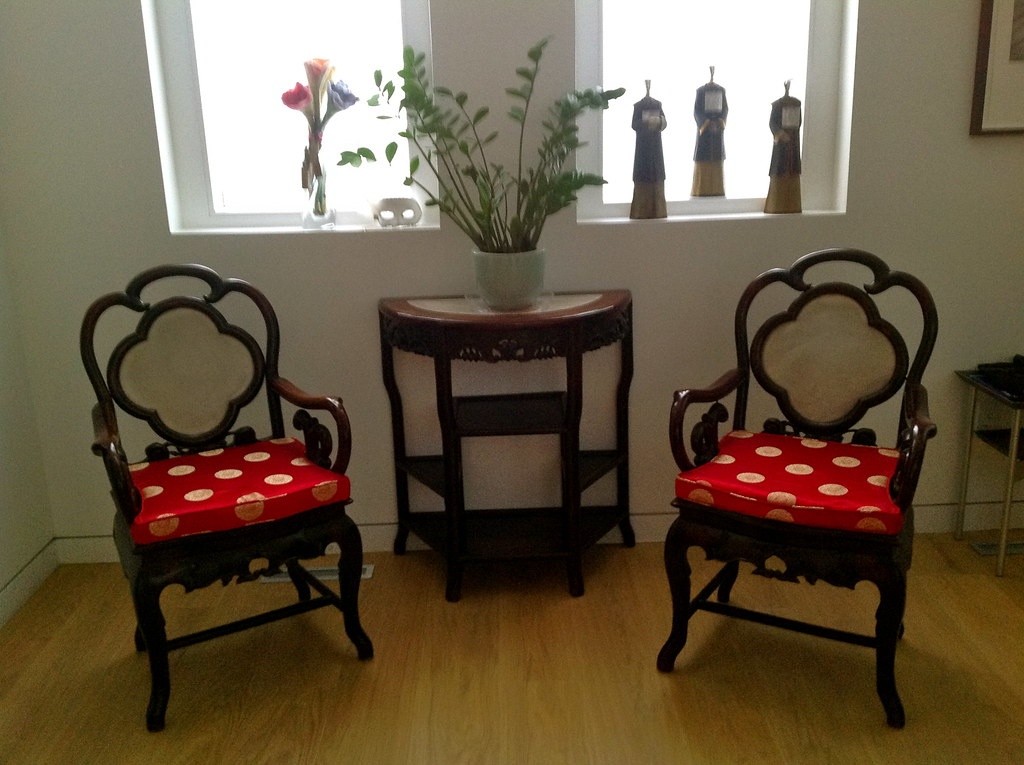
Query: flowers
[278,55,360,216]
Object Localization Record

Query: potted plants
[334,35,628,309]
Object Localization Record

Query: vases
[304,157,337,228]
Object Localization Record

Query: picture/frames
[967,0,1024,136]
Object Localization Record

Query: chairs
[77,263,375,733]
[657,246,941,727]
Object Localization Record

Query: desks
[378,286,640,604]
[957,370,1024,577]
